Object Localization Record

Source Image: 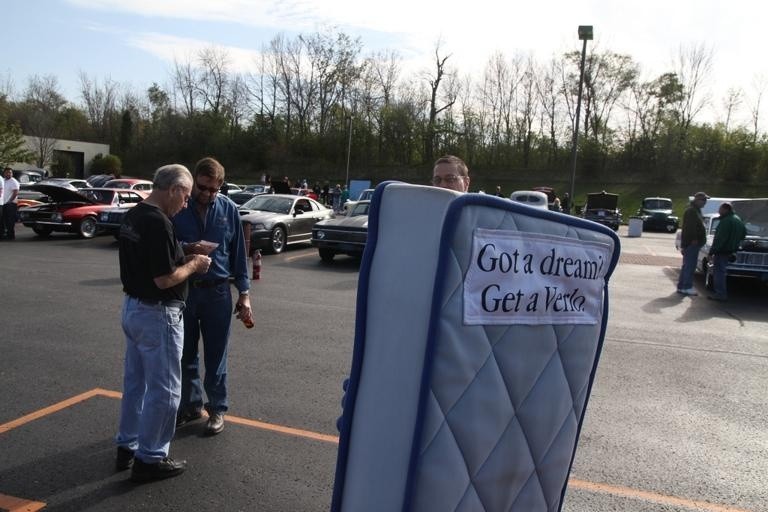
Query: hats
[694,192,710,199]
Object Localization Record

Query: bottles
[234,302,255,329]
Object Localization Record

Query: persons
[265,173,348,212]
[677,192,710,296]
[552,197,564,212]
[707,203,747,301]
[117,164,212,482]
[493,185,505,198]
[431,155,470,193]
[173,158,252,434]
[0,175,5,240]
[3,168,21,240]
[220,181,229,196]
[560,192,574,215]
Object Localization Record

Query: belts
[138,297,182,308]
[188,278,223,289]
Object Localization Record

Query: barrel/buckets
[627,218,643,238]
[240,222,250,256]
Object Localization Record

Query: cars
[311,199,371,264]
[0,168,154,239]
[534,186,562,212]
[221,180,318,205]
[511,190,548,213]
[345,187,375,202]
[673,195,767,289]
[580,190,623,231]
[238,193,335,255]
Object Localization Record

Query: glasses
[429,176,465,184]
[195,180,221,192]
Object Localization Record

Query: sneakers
[708,294,720,299]
[116,448,187,483]
[203,412,223,433]
[677,287,698,295]
[176,411,202,426]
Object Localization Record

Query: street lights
[564,23,595,211]
[346,114,356,186]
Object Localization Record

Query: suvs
[636,196,681,233]
